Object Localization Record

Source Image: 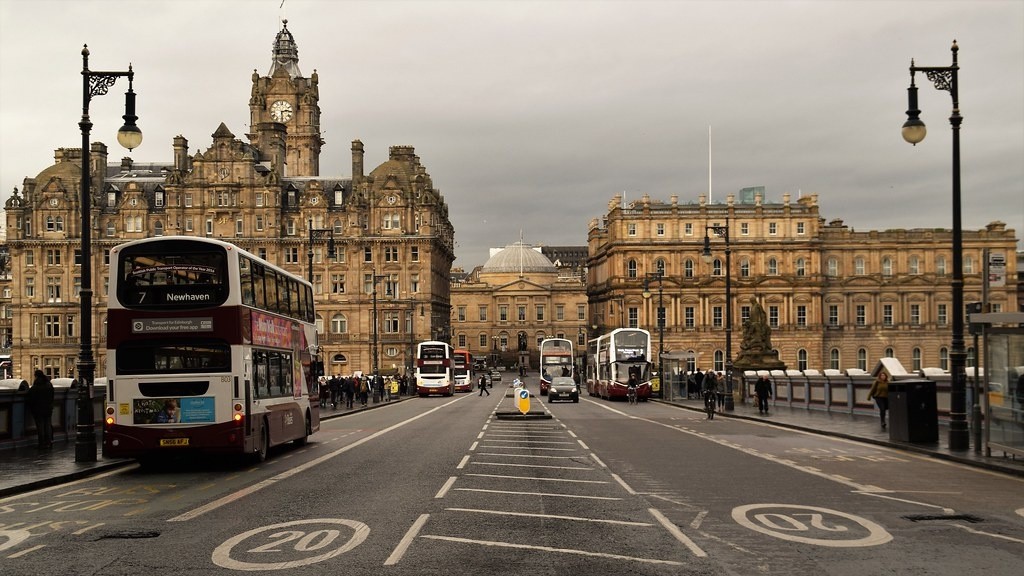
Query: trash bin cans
[888,379,939,443]
[651,371,659,396]
[388,383,400,400]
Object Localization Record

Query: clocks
[268,100,294,123]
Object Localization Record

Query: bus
[586,328,652,402]
[540,338,574,394]
[103,236,325,466]
[454,350,476,392]
[416,341,457,397]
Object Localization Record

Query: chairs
[132,268,310,323]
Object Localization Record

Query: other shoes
[479,394,482,396]
[627,394,629,396]
[880,423,887,427]
[487,394,490,396]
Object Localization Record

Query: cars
[478,375,492,388]
[487,367,501,381]
[497,365,506,372]
[547,377,580,403]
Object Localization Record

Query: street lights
[409,297,424,396]
[643,265,663,397]
[609,299,623,328]
[308,215,336,284]
[75,44,142,460]
[592,309,605,335]
[901,40,970,449]
[373,268,392,403]
[702,216,734,410]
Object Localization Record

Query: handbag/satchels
[326,392,329,398]
[872,381,879,398]
[752,393,759,407]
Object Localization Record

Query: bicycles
[704,390,720,420]
[627,385,638,405]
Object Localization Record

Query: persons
[154,399,177,423]
[320,371,416,411]
[574,372,582,394]
[626,374,640,405]
[478,374,490,397]
[867,372,890,432]
[26,370,55,459]
[678,367,726,420]
[754,374,773,416]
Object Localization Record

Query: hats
[708,369,714,373]
[631,373,636,376]
[763,375,768,379]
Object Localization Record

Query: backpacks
[361,382,367,392]
